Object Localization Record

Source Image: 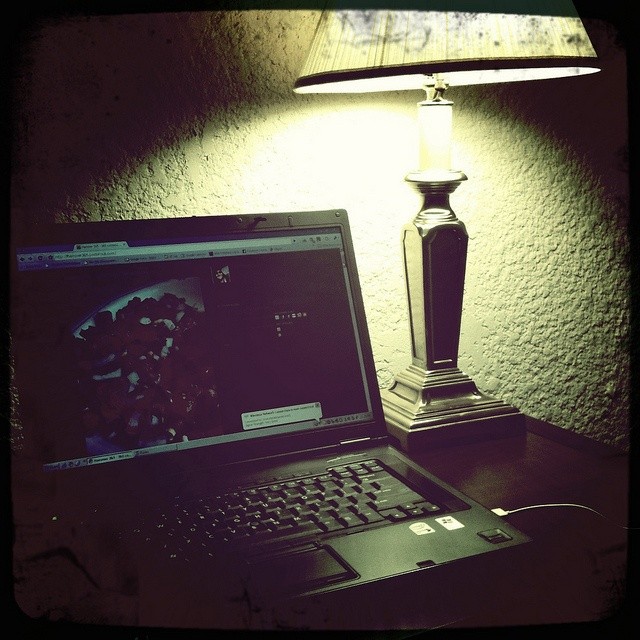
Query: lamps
[291,7,602,453]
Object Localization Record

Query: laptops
[2,208,534,635]
